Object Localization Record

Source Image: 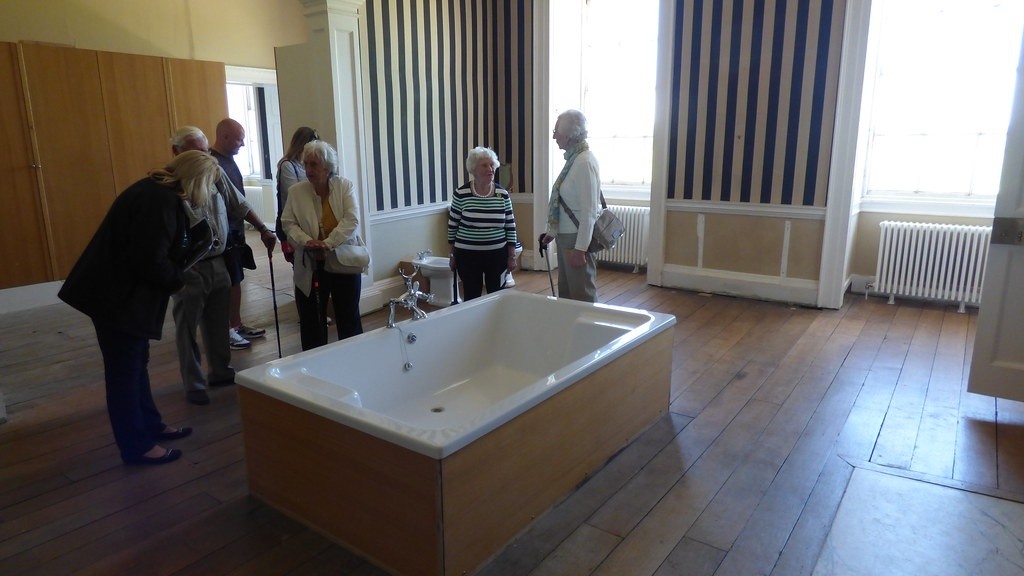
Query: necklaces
[471,179,494,197]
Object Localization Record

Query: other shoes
[190,390,210,405]
[155,424,193,442]
[207,376,234,387]
[327,316,332,328]
[121,444,182,465]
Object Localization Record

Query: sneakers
[235,323,266,338]
[229,328,251,350]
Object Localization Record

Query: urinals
[412,254,462,307]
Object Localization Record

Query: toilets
[483,242,523,287]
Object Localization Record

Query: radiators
[875,221,993,312]
[595,204,652,274]
[241,186,262,230]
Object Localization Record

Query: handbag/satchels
[587,210,625,252]
[276,214,294,263]
[222,247,244,283]
[325,244,370,274]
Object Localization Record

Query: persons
[539,110,599,304]
[446,147,517,302]
[209,118,267,351]
[171,126,277,407]
[275,126,363,353]
[57,149,220,467]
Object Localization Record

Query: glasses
[553,129,558,133]
[177,146,211,155]
[311,128,320,138]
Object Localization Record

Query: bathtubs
[234,287,677,576]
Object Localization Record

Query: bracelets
[261,229,276,236]
[448,252,454,257]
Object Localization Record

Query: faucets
[385,298,428,329]
[417,247,433,260]
